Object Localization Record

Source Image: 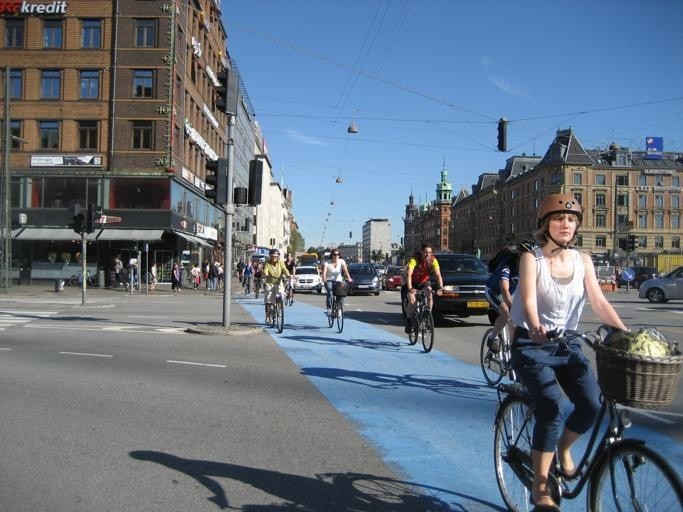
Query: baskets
[596,342,682,411]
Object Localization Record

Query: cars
[292,266,321,294]
[347,263,406,295]
[401,253,499,325]
[639,266,683,303]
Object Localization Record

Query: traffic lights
[87,203,101,233]
[627,235,639,250]
[205,159,227,204]
[213,69,238,115]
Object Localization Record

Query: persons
[113,257,130,292]
[283,252,297,302]
[190,260,224,291]
[486,240,539,353]
[150,262,158,291]
[262,249,291,322]
[405,243,444,334]
[507,194,629,512]
[172,263,182,292]
[236,259,264,294]
[322,249,352,319]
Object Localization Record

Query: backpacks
[488,246,519,273]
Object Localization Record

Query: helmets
[519,238,540,250]
[537,194,583,223]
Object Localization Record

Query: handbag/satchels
[603,327,669,356]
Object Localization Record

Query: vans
[615,267,659,290]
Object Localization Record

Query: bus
[297,252,318,266]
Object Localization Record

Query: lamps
[320,110,358,242]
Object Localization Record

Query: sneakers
[487,335,500,353]
[404,317,412,333]
[529,494,560,511]
[562,461,575,478]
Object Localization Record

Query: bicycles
[405,290,436,353]
[240,275,297,333]
[324,280,349,333]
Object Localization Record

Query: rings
[530,331,537,336]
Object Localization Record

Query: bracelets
[409,288,412,293]
